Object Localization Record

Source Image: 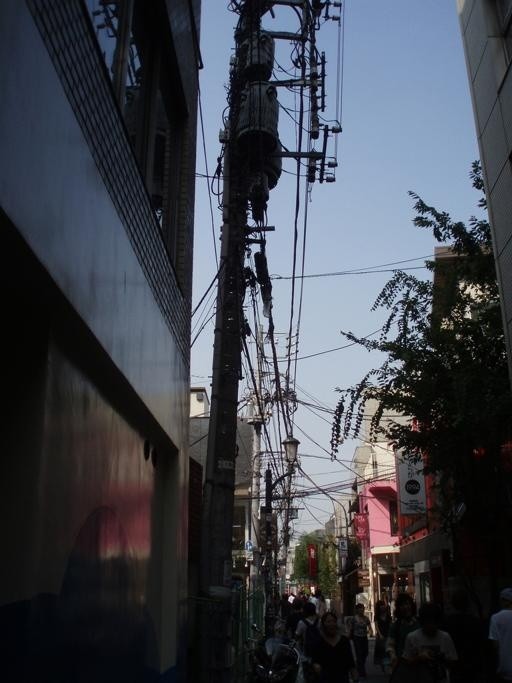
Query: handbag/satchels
[389,658,436,683]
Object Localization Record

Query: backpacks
[302,616,322,658]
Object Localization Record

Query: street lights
[263,433,300,638]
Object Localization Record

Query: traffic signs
[245,540,254,561]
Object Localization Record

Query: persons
[262,586,460,682]
[486,587,511,682]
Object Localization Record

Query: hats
[499,588,512,601]
